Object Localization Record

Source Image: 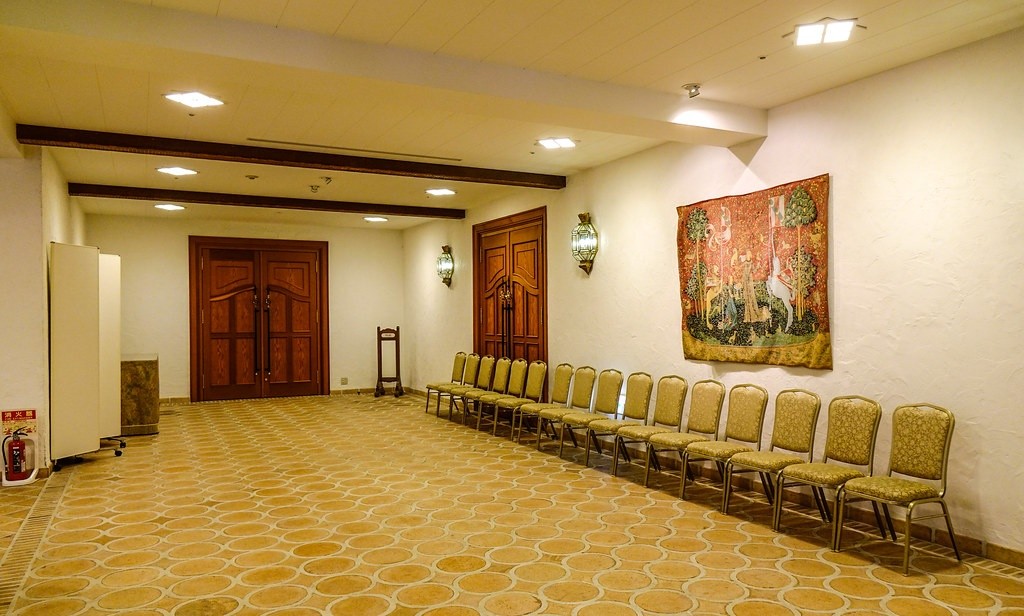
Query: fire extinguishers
[2,426,28,481]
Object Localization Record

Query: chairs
[424,351,965,577]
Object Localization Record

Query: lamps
[436,245,455,287]
[682,83,702,99]
[570,212,599,275]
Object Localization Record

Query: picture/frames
[675,171,834,368]
